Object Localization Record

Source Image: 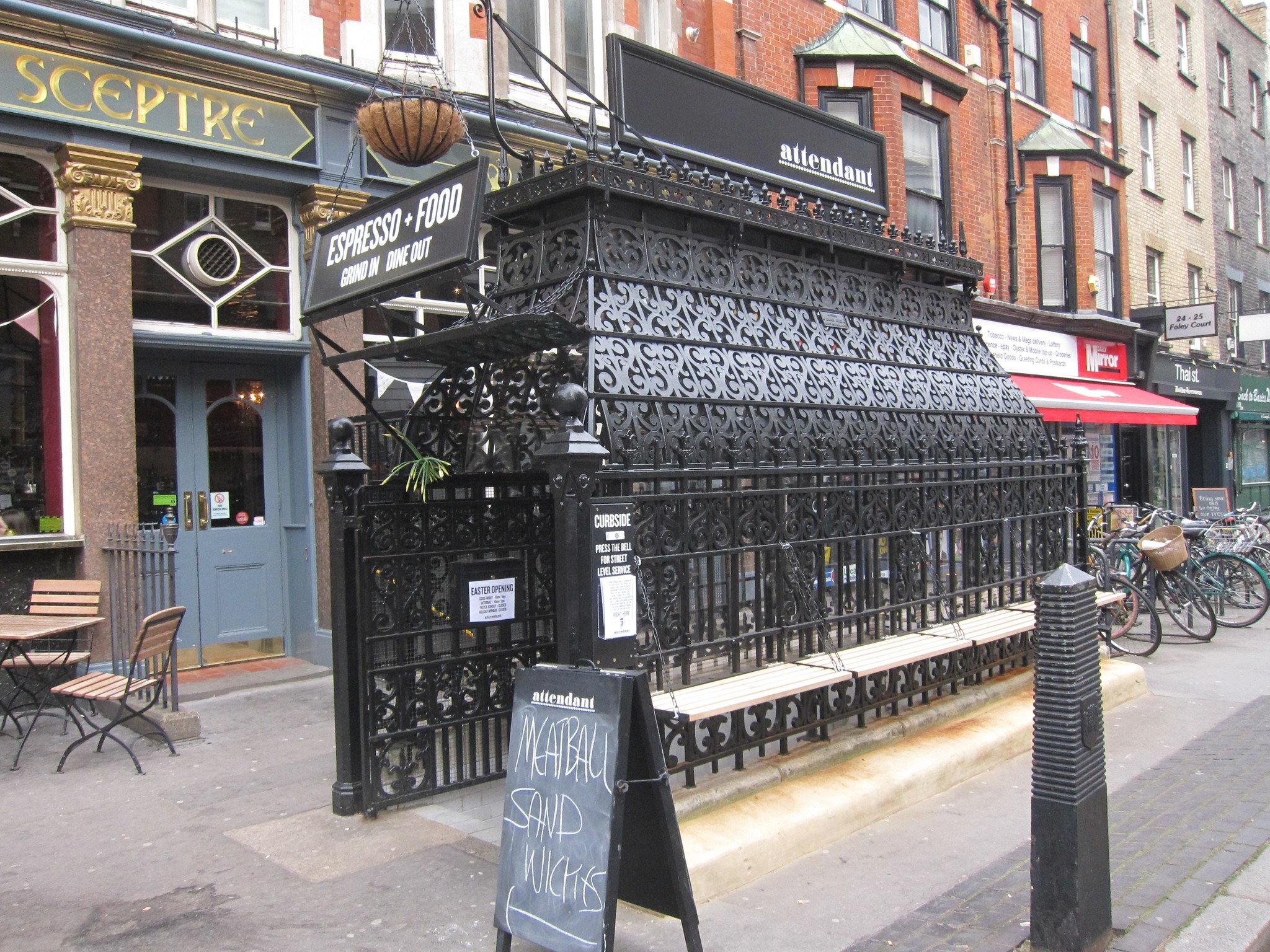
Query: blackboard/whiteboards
[1192,488,1230,520]
[494,663,703,952]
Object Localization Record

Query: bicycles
[1083,498,1270,657]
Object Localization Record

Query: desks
[0,615,106,770]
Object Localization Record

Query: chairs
[0,579,102,737]
[52,606,187,775]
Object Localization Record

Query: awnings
[1005,374,1199,429]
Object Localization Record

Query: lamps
[1174,357,1269,373]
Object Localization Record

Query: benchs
[635,506,1128,790]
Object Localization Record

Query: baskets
[1137,525,1188,571]
[1204,514,1260,553]
